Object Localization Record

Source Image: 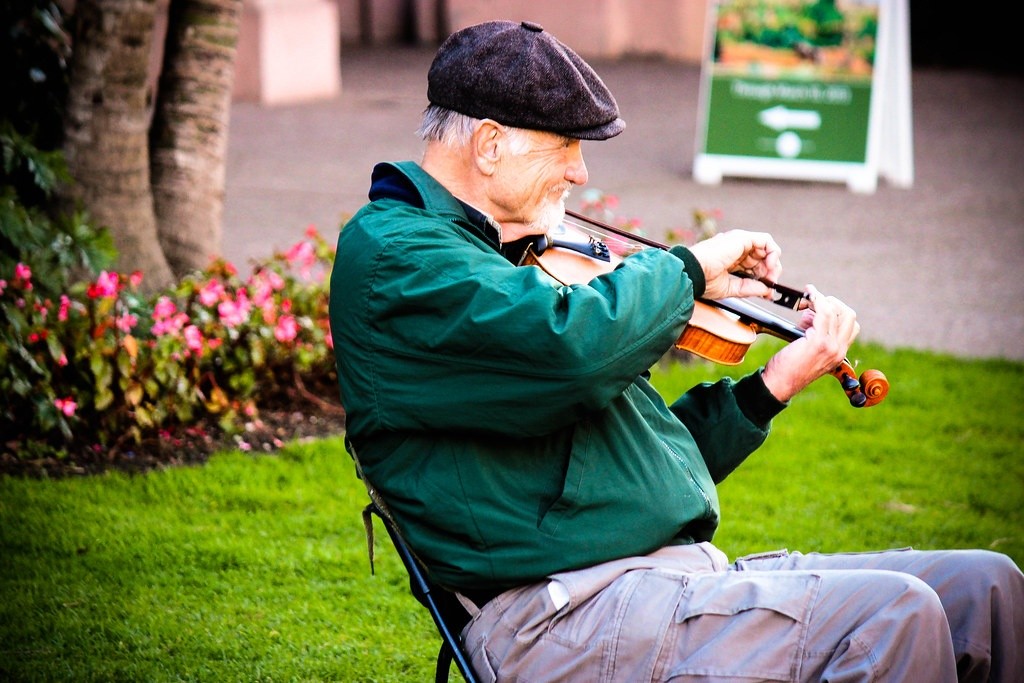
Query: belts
[440,580,517,636]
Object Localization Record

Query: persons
[328,20,1024,683]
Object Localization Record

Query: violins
[516,226,890,407]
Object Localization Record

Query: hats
[427,21,626,142]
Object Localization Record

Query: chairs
[344,429,476,683]
